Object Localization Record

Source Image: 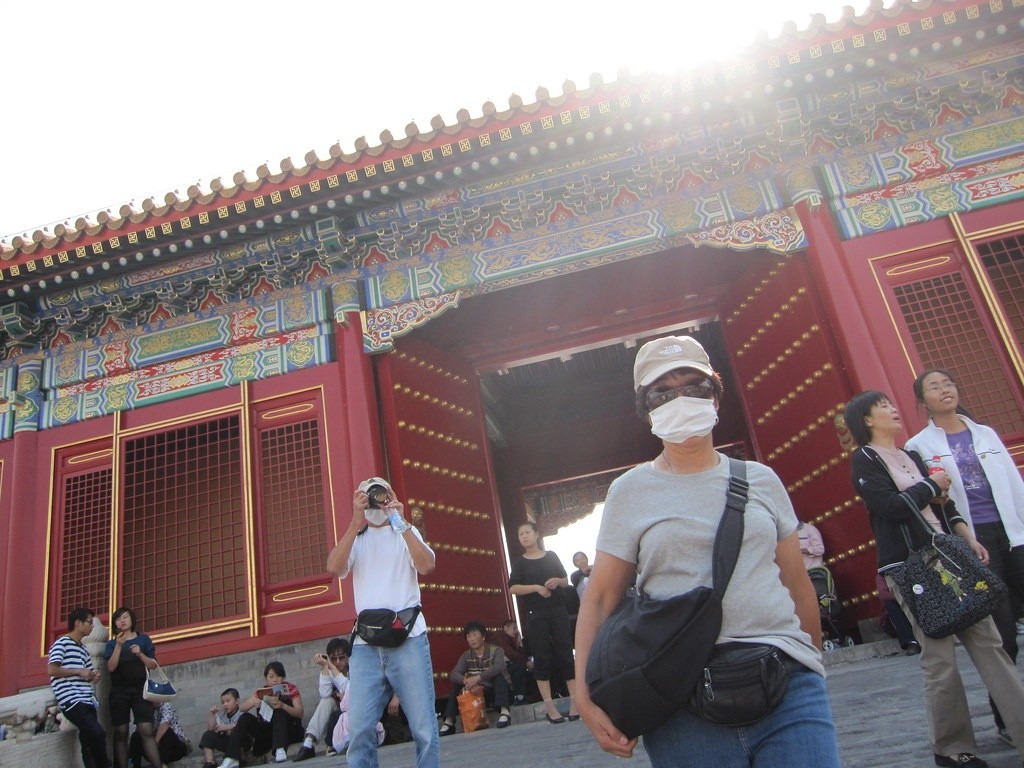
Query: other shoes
[275,747,288,762]
[217,757,240,768]
[292,746,316,761]
[934,751,988,768]
[906,641,921,655]
[202,761,218,768]
[326,746,337,757]
[497,713,511,728]
[439,723,456,737]
[546,712,565,723]
[999,727,1017,748]
[568,713,581,721]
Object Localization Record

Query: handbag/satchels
[586,586,726,740]
[888,491,1008,638]
[354,606,422,649]
[686,643,793,726]
[143,659,177,703]
[456,685,490,734]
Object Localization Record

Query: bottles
[383,499,406,533]
[928,455,946,504]
[44,715,54,734]
[214,709,226,736]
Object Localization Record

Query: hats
[358,477,390,494]
[634,335,712,393]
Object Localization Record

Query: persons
[438,622,513,735]
[289,637,350,762]
[198,661,305,768]
[47,608,112,768]
[796,518,842,633]
[842,369,1024,768]
[572,336,843,768]
[129,701,194,768]
[326,476,441,768]
[488,520,594,723]
[104,607,164,768]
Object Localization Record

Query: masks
[649,396,720,444]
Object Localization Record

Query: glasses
[645,377,718,411]
[83,619,94,625]
[922,382,956,395]
[329,654,349,662]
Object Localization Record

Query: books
[256,683,290,702]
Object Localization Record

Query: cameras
[364,487,390,509]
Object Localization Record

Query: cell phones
[115,628,121,634]
